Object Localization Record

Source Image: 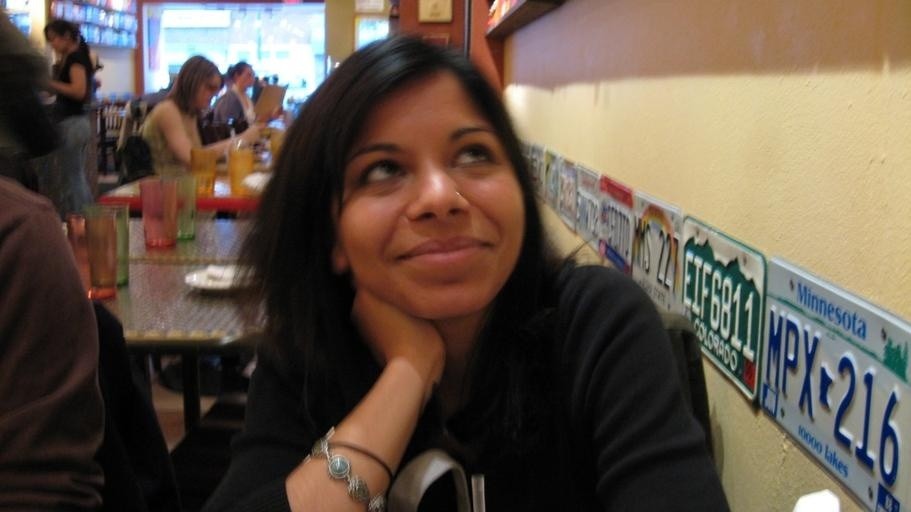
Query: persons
[208,61,259,141]
[139,56,271,177]
[0,11,107,508]
[40,16,98,223]
[200,32,732,512]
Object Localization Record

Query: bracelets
[329,438,397,481]
[309,427,392,511]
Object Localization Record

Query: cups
[189,147,216,194]
[227,135,253,195]
[269,128,285,160]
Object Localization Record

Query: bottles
[63,200,131,302]
[137,173,196,251]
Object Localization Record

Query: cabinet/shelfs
[49,3,139,50]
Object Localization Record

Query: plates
[182,267,257,293]
[241,170,269,191]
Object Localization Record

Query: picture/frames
[415,32,450,47]
[416,0,456,24]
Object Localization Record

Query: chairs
[96,98,124,171]
